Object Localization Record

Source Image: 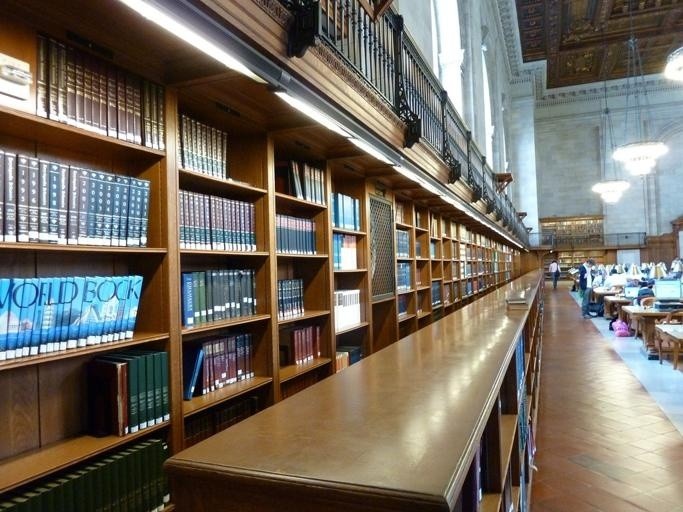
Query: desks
[568,261,683,369]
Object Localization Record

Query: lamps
[591,71,629,204]
[612,0,667,175]
[664,47,683,81]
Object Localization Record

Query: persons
[580,258,595,318]
[591,256,683,306]
[548,260,562,290]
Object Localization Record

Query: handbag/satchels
[611,319,630,337]
[554,270,560,277]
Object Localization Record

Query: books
[396,200,512,337]
[332,193,365,371]
[1,24,171,511]
[178,107,264,446]
[478,333,534,512]
[276,148,326,398]
